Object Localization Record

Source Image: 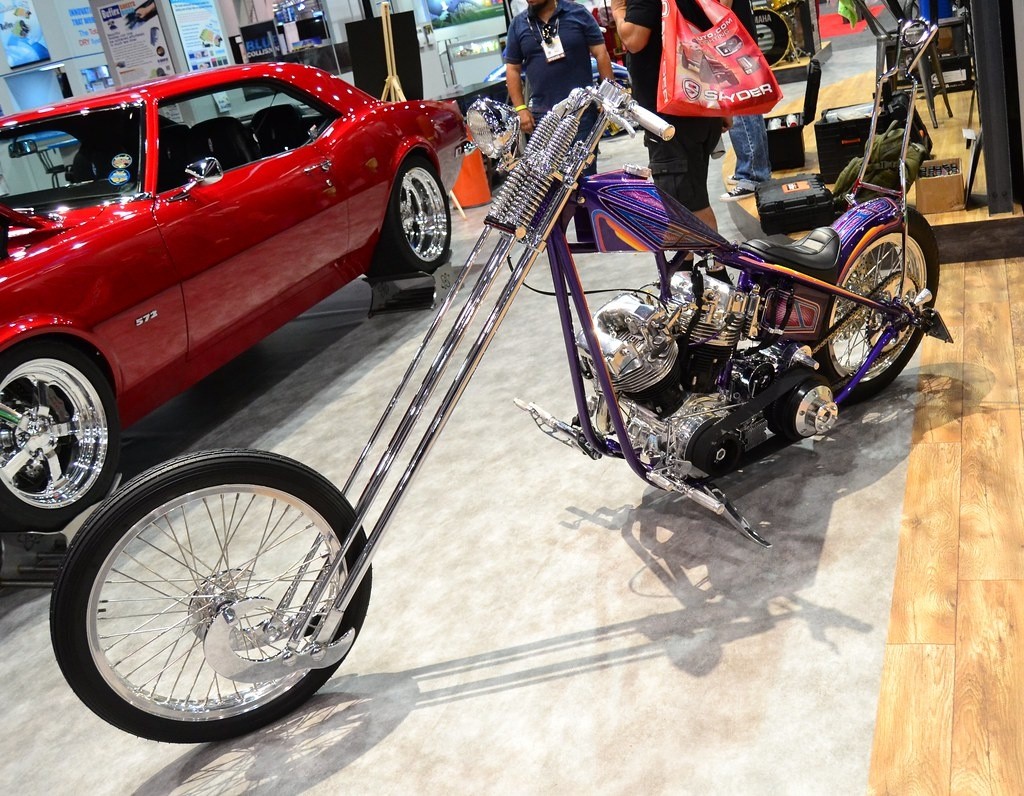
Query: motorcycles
[49,17,955,746]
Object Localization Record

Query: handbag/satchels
[656,0,784,118]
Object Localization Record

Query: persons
[610,0,733,284]
[717,0,771,202]
[504,0,614,174]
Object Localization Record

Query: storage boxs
[763,59,822,172]
[926,19,970,93]
[914,158,966,215]
[814,90,933,185]
[753,173,837,235]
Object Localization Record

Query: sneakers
[728,175,741,185]
[720,187,757,201]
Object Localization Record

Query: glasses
[542,23,553,47]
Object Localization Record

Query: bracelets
[515,105,526,111]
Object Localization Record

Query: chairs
[72,103,308,187]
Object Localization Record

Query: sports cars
[0,60,475,536]
